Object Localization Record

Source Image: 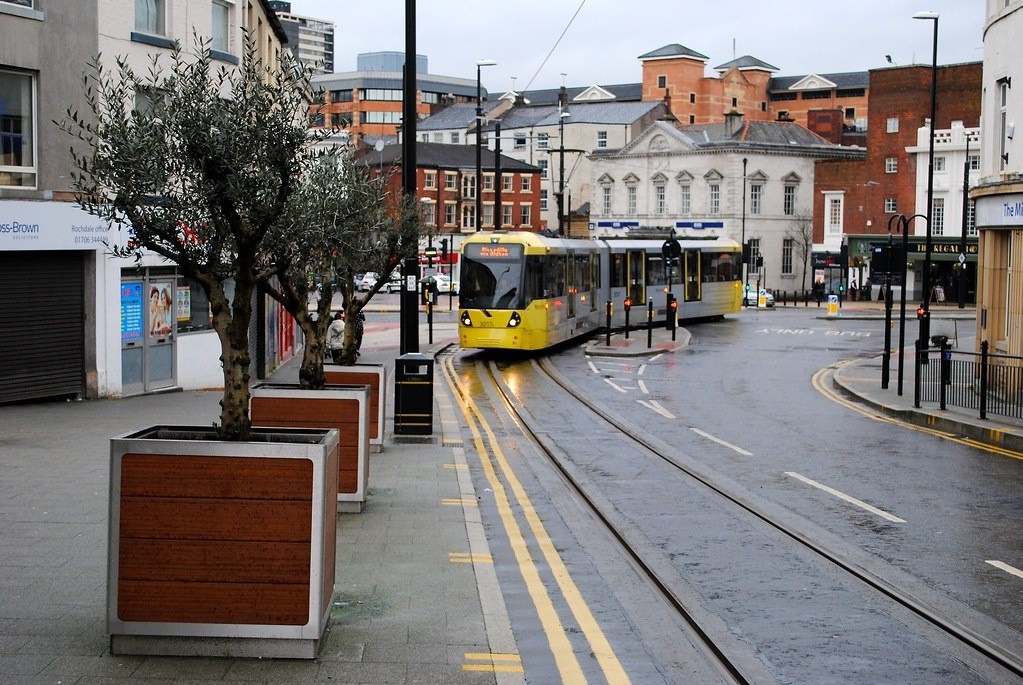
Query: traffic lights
[439,239,448,260]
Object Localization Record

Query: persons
[326,312,345,363]
[150,287,172,333]
[324,310,365,359]
[866,277,872,301]
[850,279,857,301]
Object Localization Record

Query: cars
[741,284,775,307]
[306,270,402,295]
[423,274,460,297]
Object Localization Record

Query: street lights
[957,130,972,307]
[474,60,499,232]
[912,9,941,366]
[558,112,572,239]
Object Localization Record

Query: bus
[457,228,744,357]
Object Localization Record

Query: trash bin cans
[931,335,952,385]
[393,352,436,435]
